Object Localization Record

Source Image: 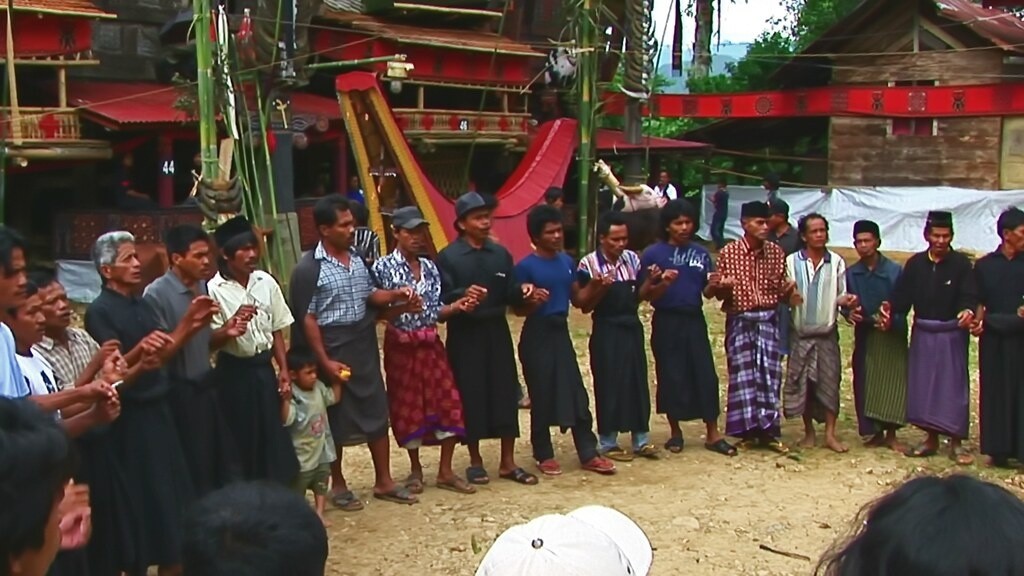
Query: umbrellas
[157,9,196,45]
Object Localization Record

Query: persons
[812,474,1024,576]
[279,346,351,528]
[346,173,368,226]
[182,478,328,576]
[839,220,909,451]
[576,212,663,462]
[640,199,738,456]
[290,195,420,512]
[206,214,301,487]
[143,224,247,493]
[969,205,1024,468]
[714,200,797,454]
[0,213,167,576]
[435,192,539,486]
[783,212,858,454]
[113,169,154,210]
[475,504,652,576]
[879,210,976,466]
[526,171,802,259]
[511,204,617,476]
[84,231,214,567]
[371,206,477,493]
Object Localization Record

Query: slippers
[633,444,661,456]
[536,460,562,475]
[406,474,422,494]
[330,489,364,511]
[582,458,616,471]
[735,438,757,450]
[704,436,737,455]
[373,483,419,504]
[499,467,539,485]
[665,438,683,453]
[466,466,490,484]
[436,475,476,494]
[904,442,937,457]
[602,448,633,460]
[949,446,975,464]
[759,439,790,452]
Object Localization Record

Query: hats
[453,192,498,234]
[475,504,654,576]
[214,214,250,248]
[393,206,430,230]
[927,211,953,228]
[741,201,771,218]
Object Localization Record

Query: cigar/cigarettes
[253,298,256,309]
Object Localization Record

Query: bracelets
[963,308,974,317]
[450,303,453,313]
